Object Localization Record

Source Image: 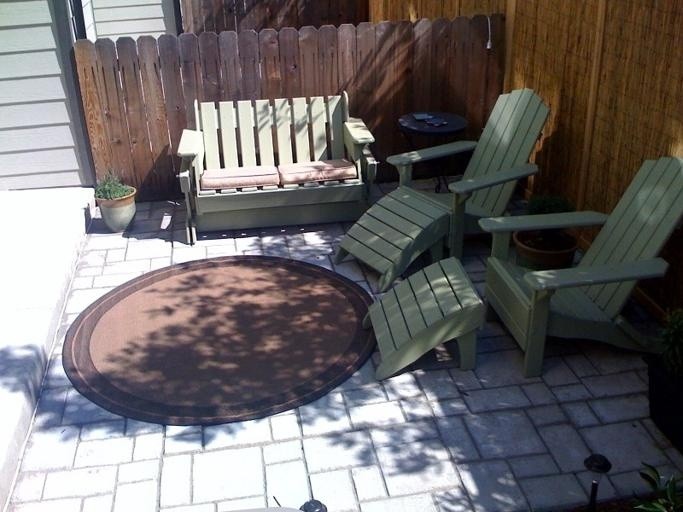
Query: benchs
[171,89,380,244]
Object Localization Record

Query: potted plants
[91,172,139,234]
[640,309,681,450]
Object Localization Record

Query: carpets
[59,252,381,427]
